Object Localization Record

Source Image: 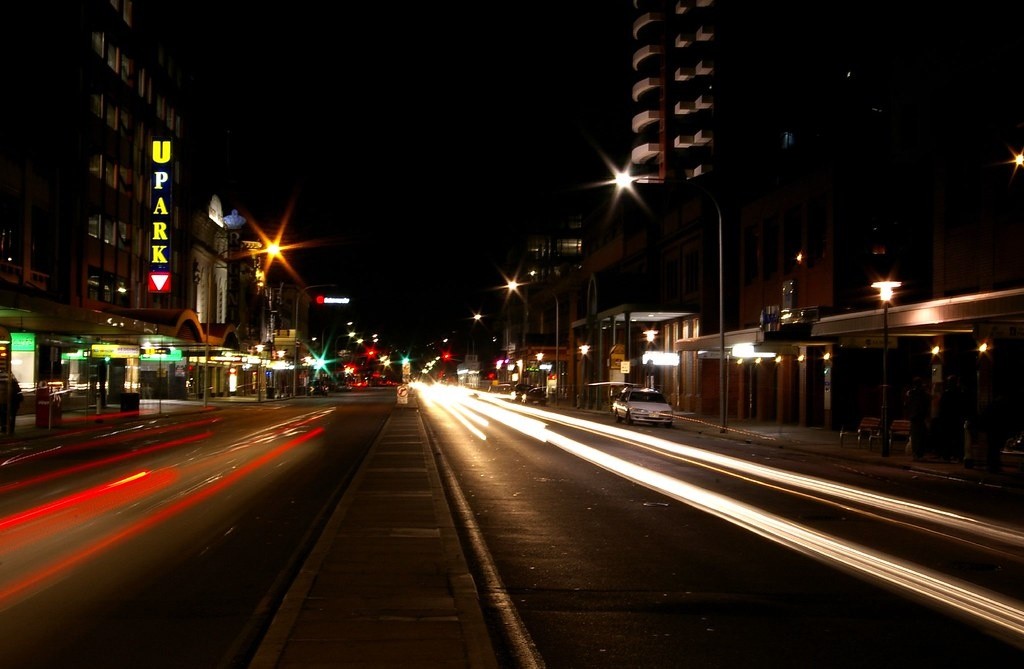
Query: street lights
[202,243,282,405]
[611,175,729,432]
[293,283,336,398]
[507,279,560,403]
[870,281,903,455]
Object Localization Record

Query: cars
[610,387,674,429]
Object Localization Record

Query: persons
[307,379,327,397]
[0,371,21,435]
[904,375,971,463]
